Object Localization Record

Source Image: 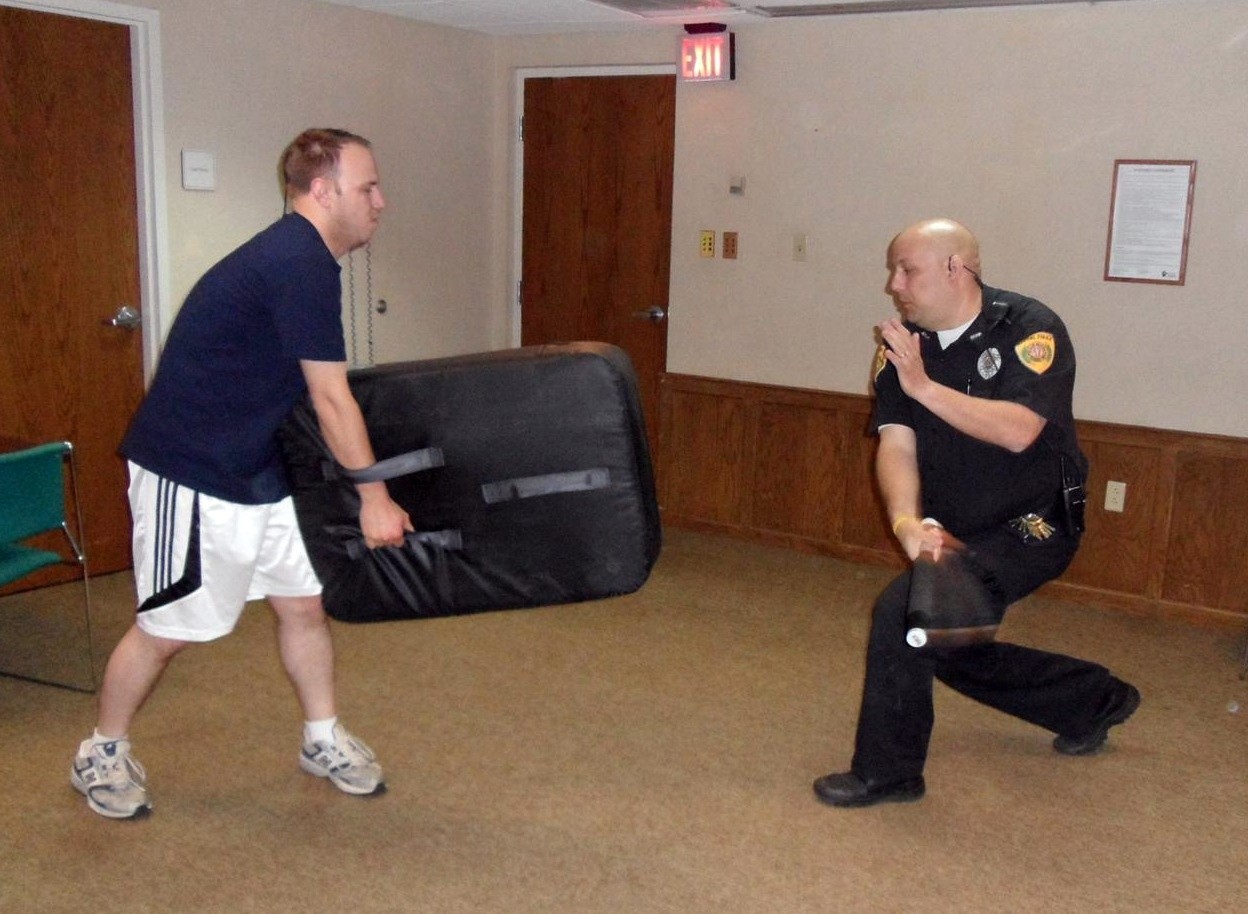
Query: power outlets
[1103,480,1127,513]
[699,230,716,257]
[723,231,738,258]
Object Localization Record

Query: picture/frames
[1104,157,1199,285]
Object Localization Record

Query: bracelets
[892,516,919,536]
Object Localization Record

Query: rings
[898,352,906,360]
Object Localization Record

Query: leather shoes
[814,772,925,808]
[1053,676,1140,756]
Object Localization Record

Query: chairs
[0,438,99,696]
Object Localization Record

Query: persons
[813,217,1140,806]
[69,128,414,821]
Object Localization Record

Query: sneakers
[298,722,388,798]
[70,738,155,822]
